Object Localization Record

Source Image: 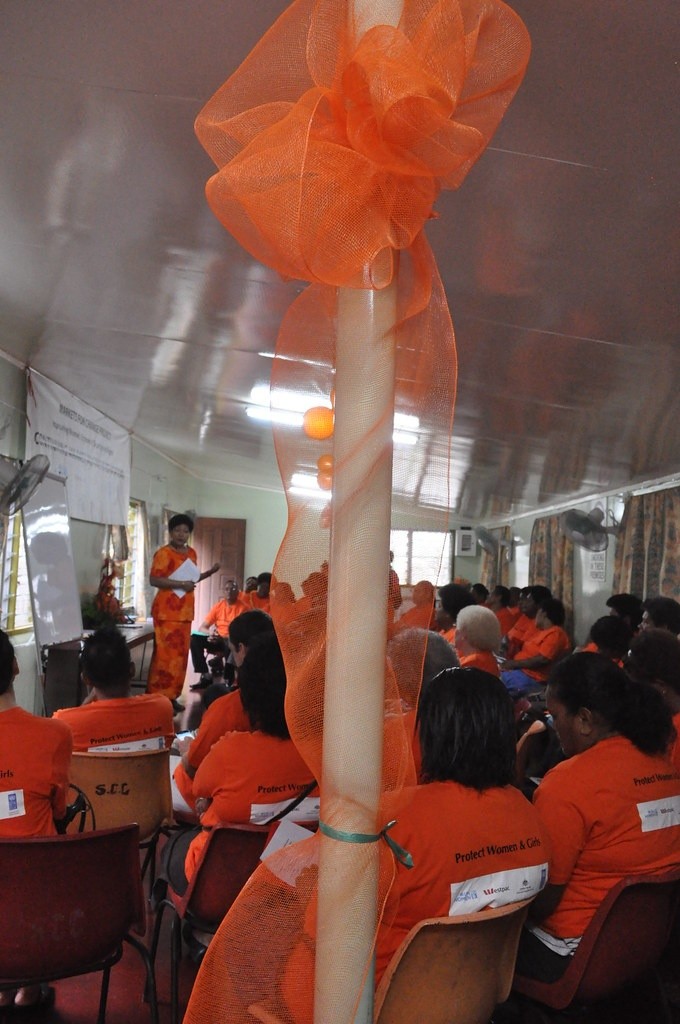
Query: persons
[159,548,679,1024]
[51,628,177,752]
[0,629,74,1024]
[149,512,220,712]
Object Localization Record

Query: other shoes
[190,677,213,689]
[170,699,185,717]
[0,987,55,1024]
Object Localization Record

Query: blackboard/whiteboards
[15,458,85,649]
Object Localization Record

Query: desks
[45,622,157,718]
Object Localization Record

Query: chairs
[141,821,321,1024]
[64,749,173,906]
[370,886,538,1024]
[515,715,555,788]
[513,870,680,1024]
[0,819,160,1024]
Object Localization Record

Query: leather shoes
[182,922,208,963]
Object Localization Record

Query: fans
[474,525,514,564]
[559,506,625,553]
[0,453,50,516]
